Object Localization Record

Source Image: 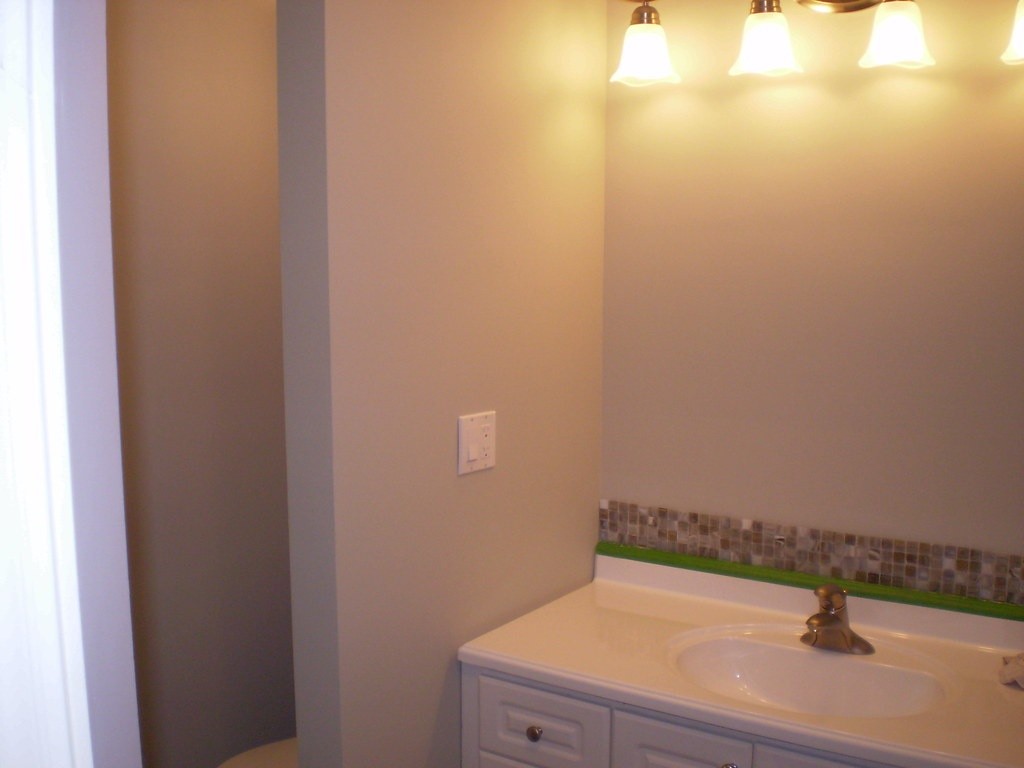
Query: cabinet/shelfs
[476,675,863,768]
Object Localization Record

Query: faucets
[800,585,876,656]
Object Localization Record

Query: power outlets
[458,410,495,477]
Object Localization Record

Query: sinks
[673,636,958,723]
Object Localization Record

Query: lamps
[610,0,1024,85]
